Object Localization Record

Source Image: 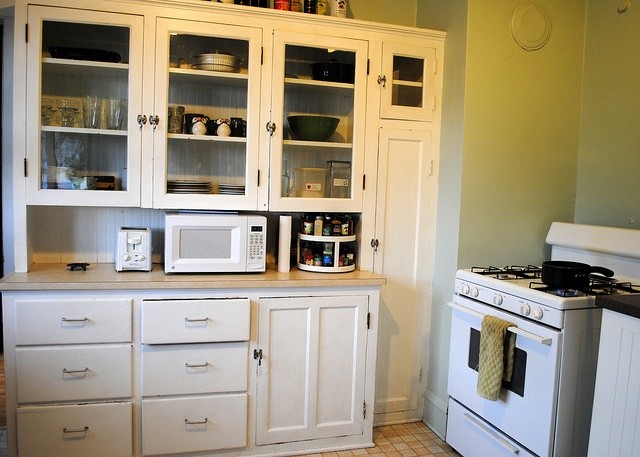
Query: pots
[192,49,241,72]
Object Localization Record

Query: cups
[82,96,101,129]
[105,99,125,130]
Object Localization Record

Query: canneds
[306,255,314,265]
[342,215,353,235]
[314,258,322,266]
[323,243,333,252]
[343,257,349,266]
[331,220,341,236]
[339,257,344,266]
[303,215,314,235]
[323,251,332,266]
[322,217,332,235]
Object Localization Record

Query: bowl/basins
[287,115,340,140]
[309,60,354,83]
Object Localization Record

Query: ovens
[443,297,604,456]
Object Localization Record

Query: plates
[49,47,121,62]
[44,166,76,188]
[218,182,248,196]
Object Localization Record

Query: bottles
[332,220,342,234]
[229,115,244,136]
[330,0,348,19]
[324,215,333,235]
[302,216,313,235]
[314,216,323,236]
[167,177,213,194]
[323,243,334,267]
[303,254,313,265]
[342,220,350,234]
[347,253,355,263]
[304,0,316,14]
[312,256,322,265]
[343,255,348,265]
[337,256,344,268]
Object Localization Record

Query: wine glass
[55,97,81,126]
[41,98,57,126]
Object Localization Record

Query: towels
[477,315,517,401]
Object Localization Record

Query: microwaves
[163,212,267,277]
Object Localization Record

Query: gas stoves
[453,259,614,329]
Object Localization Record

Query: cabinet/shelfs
[270,11,369,213]
[369,20,447,121]
[0,282,137,457]
[137,283,251,456]
[25,2,262,213]
[587,299,639,456]
[358,122,441,426]
[252,275,386,456]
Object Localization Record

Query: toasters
[115,225,153,273]
